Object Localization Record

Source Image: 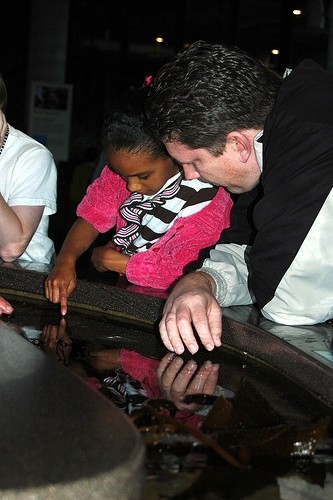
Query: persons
[0,78,59,276]
[43,73,235,318]
[145,38,333,356]
[35,317,205,434]
[154,347,233,420]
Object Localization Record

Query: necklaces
[0,124,9,155]
